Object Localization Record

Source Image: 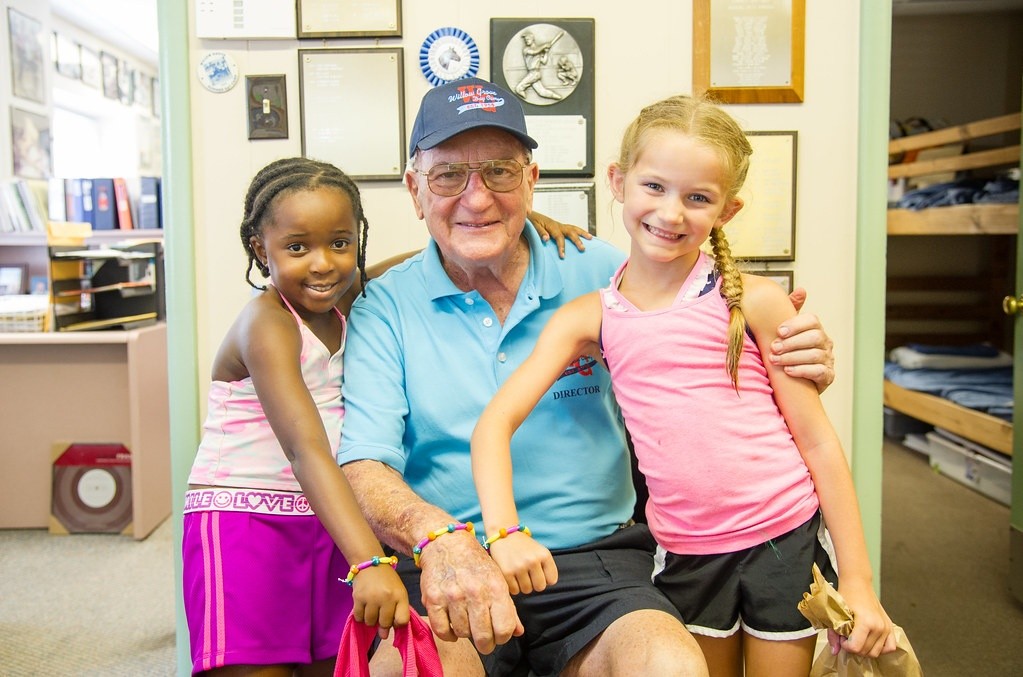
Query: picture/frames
[11,104,53,181]
[297,46,407,181]
[7,4,49,105]
[707,130,799,262]
[531,181,596,238]
[49,27,162,118]
[296,0,403,39]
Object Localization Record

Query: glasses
[411,158,528,197]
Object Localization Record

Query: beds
[886,112,1023,234]
[885,275,1013,458]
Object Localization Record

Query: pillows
[889,339,1010,368]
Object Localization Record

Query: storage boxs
[924,432,1011,508]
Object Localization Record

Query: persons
[469,95,898,677]
[183,157,591,677]
[335,77,835,677]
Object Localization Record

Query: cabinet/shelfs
[0,229,173,540]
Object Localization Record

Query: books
[0,178,48,233]
[47,176,162,229]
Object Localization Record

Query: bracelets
[412,521,476,567]
[344,556,399,586]
[483,525,532,553]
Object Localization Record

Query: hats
[408,77,540,159]
